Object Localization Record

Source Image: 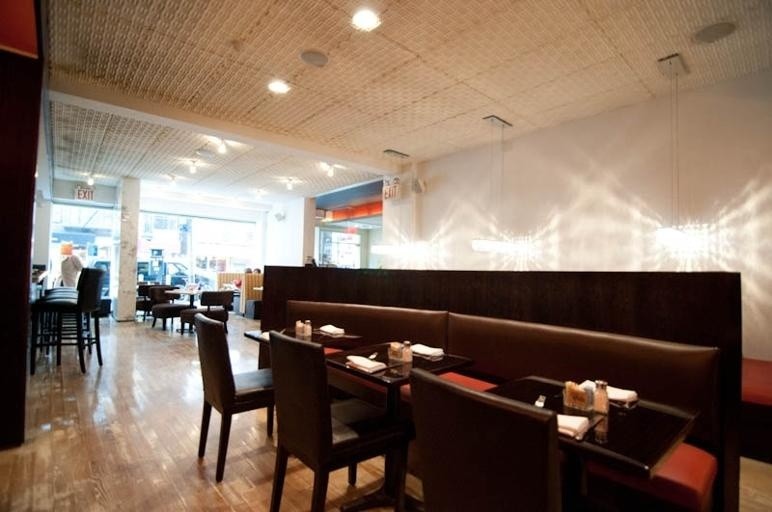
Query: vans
[93,261,211,301]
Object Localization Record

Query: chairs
[30,269,104,376]
[138,285,232,334]
[269,329,415,512]
[410,367,562,511]
[195,312,275,482]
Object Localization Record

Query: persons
[234,268,261,289]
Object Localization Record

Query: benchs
[443,313,717,512]
[281,299,447,403]
[217,271,241,313]
[234,273,264,319]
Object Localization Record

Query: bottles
[593,416,609,445]
[403,363,410,379]
[304,320,311,336]
[403,341,412,362]
[594,380,609,413]
